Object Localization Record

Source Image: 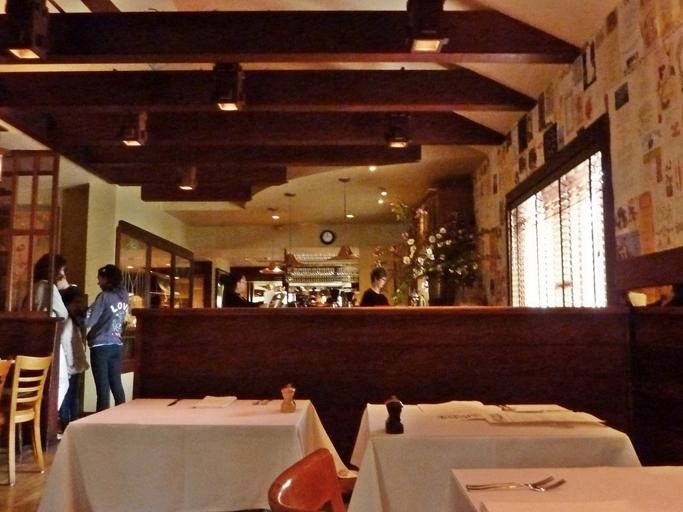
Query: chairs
[268,448,347,512]
[0,354,52,487]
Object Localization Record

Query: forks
[465,475,568,493]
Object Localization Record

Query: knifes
[167,397,184,407]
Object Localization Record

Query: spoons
[252,399,273,407]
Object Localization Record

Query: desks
[69,399,311,512]
[365,403,627,512]
[0,359,23,455]
[449,466,683,512]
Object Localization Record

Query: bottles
[384,394,404,435]
[280,383,297,413]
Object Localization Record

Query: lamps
[260,177,360,275]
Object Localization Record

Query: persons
[76,262,128,413]
[21,252,72,439]
[218,272,268,307]
[360,267,391,306]
[54,267,90,435]
